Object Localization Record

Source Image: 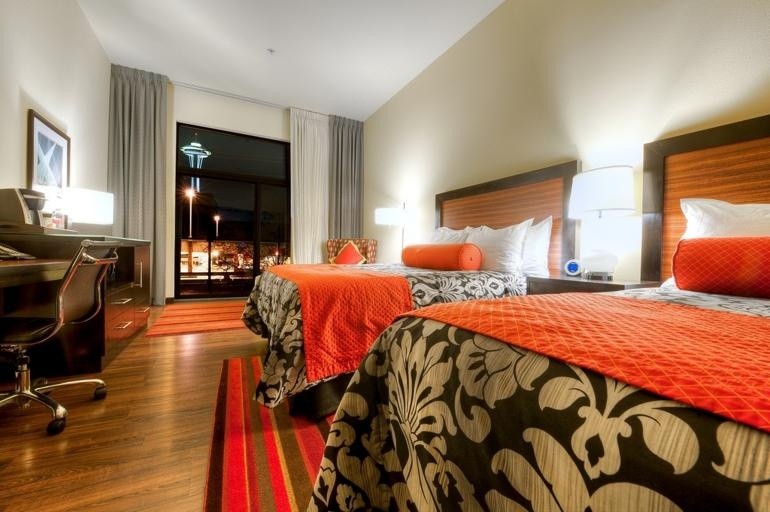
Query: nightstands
[522,271,659,296]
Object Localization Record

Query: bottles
[52,209,64,229]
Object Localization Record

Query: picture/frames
[25,107,72,234]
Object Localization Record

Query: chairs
[326,237,377,266]
[0,238,125,440]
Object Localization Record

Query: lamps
[564,163,639,224]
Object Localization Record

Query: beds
[299,111,769,512]
[234,152,583,432]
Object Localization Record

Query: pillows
[328,240,369,267]
[400,211,557,279]
[670,228,770,300]
[658,191,769,300]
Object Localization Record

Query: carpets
[140,297,251,338]
[201,353,339,512]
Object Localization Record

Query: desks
[1,248,75,292]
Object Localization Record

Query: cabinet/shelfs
[1,230,154,377]
